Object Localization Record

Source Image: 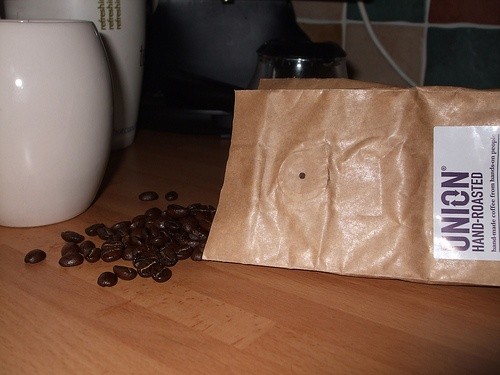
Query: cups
[0,18,115,227]
[246,41,349,89]
